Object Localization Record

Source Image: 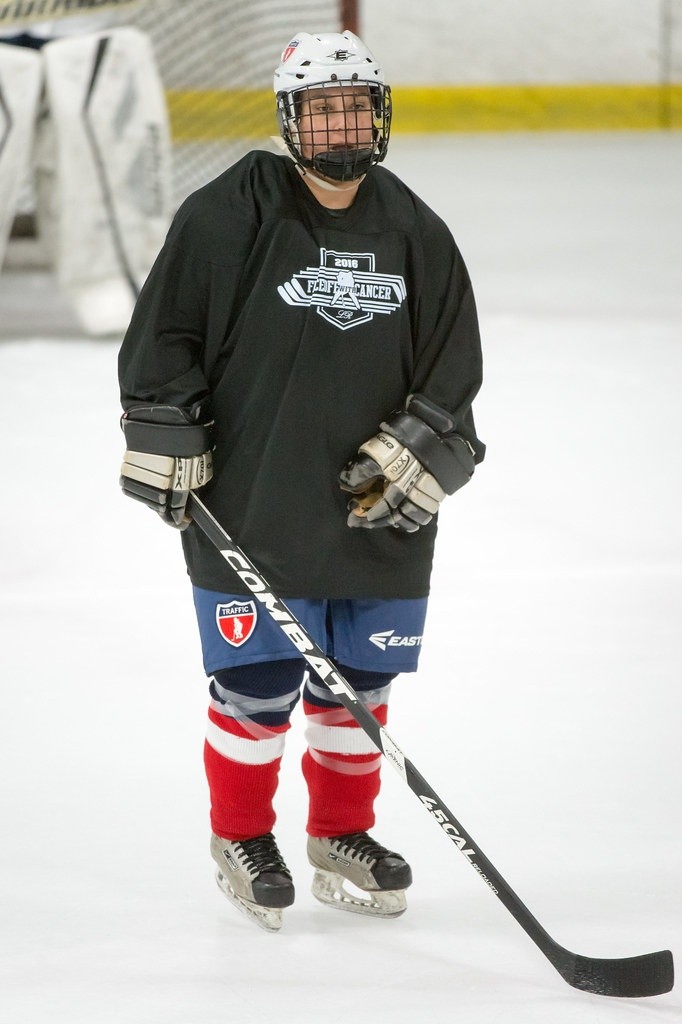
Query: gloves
[118,394,216,530]
[336,393,477,534]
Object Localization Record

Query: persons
[114,27,488,931]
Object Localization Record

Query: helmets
[272,29,383,153]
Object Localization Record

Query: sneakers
[210,831,295,933]
[307,832,412,919]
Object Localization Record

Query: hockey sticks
[185,491,676,999]
